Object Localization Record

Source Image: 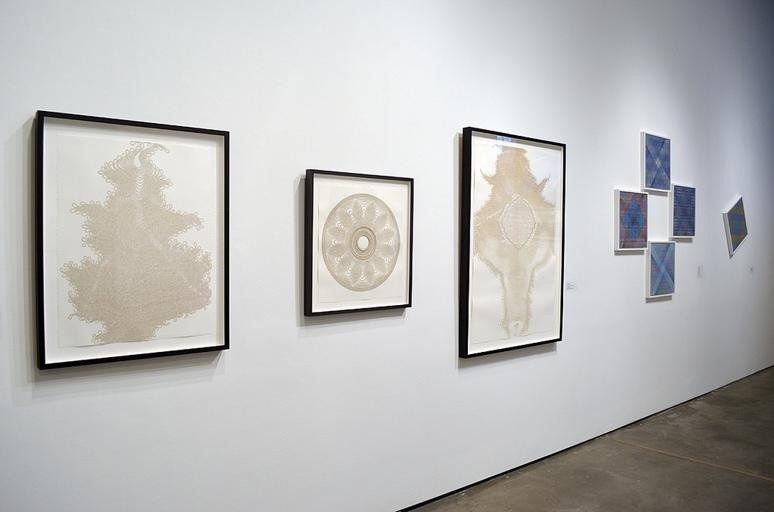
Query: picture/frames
[645,241,675,299]
[639,131,672,192]
[722,195,750,256]
[459,125,566,357]
[668,184,696,239]
[613,189,648,252]
[304,167,413,315]
[33,110,230,369]
